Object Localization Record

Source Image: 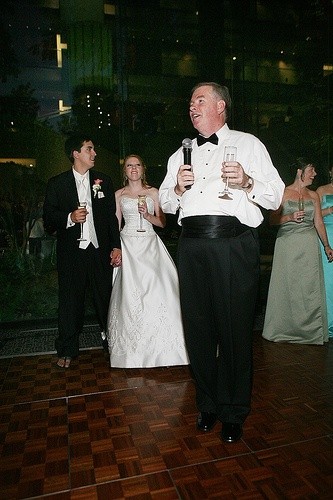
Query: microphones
[183,138,193,189]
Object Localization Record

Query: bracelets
[324,245,329,248]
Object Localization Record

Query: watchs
[242,173,253,189]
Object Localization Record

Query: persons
[314,159,333,339]
[108,153,188,369]
[259,155,333,345]
[157,81,284,443]
[43,134,122,369]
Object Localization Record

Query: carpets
[0,324,103,359]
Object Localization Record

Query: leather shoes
[222,417,244,443]
[197,410,217,431]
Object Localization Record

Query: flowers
[92,178,104,198]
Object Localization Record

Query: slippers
[56,355,73,369]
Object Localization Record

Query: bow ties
[197,133,218,146]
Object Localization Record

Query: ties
[79,176,86,194]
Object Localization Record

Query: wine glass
[299,198,304,221]
[219,146,237,195]
[137,195,146,232]
[77,202,87,241]
